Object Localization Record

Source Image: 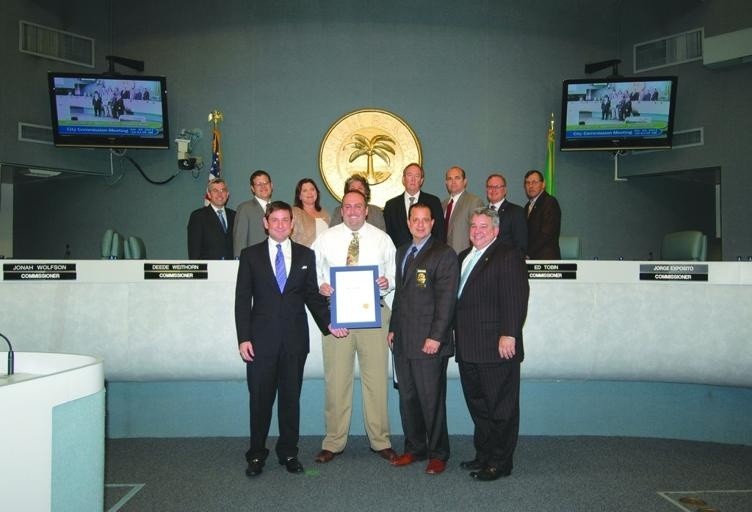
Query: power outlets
[186,156,204,171]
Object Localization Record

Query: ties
[491,205,496,210]
[458,250,481,301]
[445,200,453,231]
[346,232,359,265]
[528,201,533,218]
[276,244,287,294]
[403,247,417,284]
[217,210,226,233]
[409,197,415,206]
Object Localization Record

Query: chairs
[660,229,709,260]
[101,229,121,260]
[123,235,147,260]
[558,237,582,259]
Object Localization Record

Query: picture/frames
[329,266,381,329]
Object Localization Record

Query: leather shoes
[426,458,446,473]
[370,447,399,461]
[315,450,343,462]
[287,456,304,473]
[246,459,264,476]
[470,465,510,481]
[460,460,485,470]
[388,453,417,465]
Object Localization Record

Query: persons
[524,170,561,260]
[329,174,387,232]
[454,207,530,481]
[187,178,237,260]
[289,178,331,249]
[310,189,397,463]
[92,86,150,119]
[383,163,447,250]
[234,201,349,477]
[440,167,485,257]
[386,204,460,475]
[601,89,658,121]
[487,175,529,256]
[232,170,274,260]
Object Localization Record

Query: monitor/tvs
[49,72,169,149]
[560,76,678,152]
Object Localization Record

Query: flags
[208,130,222,181]
[545,129,555,196]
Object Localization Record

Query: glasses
[252,181,270,186]
[487,185,504,189]
[525,181,542,185]
[210,189,226,193]
